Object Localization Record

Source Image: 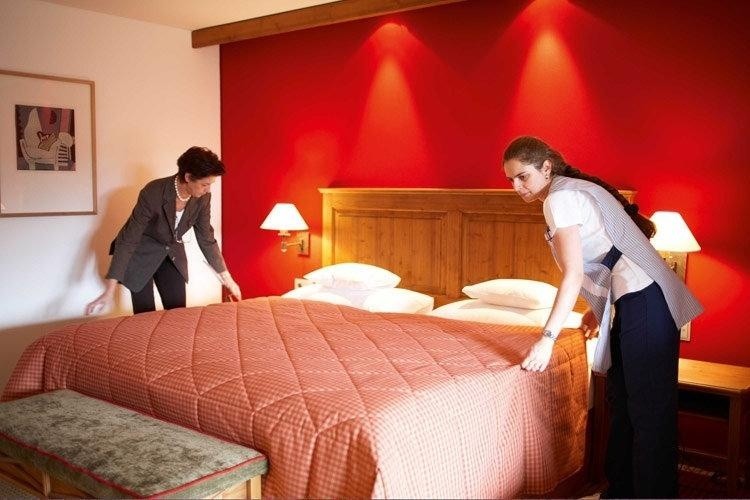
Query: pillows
[279,286,436,318]
[459,274,560,311]
[432,298,585,330]
[300,260,404,293]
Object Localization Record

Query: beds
[2,182,639,499]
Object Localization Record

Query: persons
[502,136,703,498]
[85,146,241,316]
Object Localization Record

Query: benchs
[1,386,271,500]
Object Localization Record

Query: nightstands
[669,354,750,500]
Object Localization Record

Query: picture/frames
[0,67,101,217]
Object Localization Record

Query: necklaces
[174,175,191,202]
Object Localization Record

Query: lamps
[644,210,704,286]
[259,203,312,258]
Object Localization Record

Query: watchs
[541,326,558,341]
